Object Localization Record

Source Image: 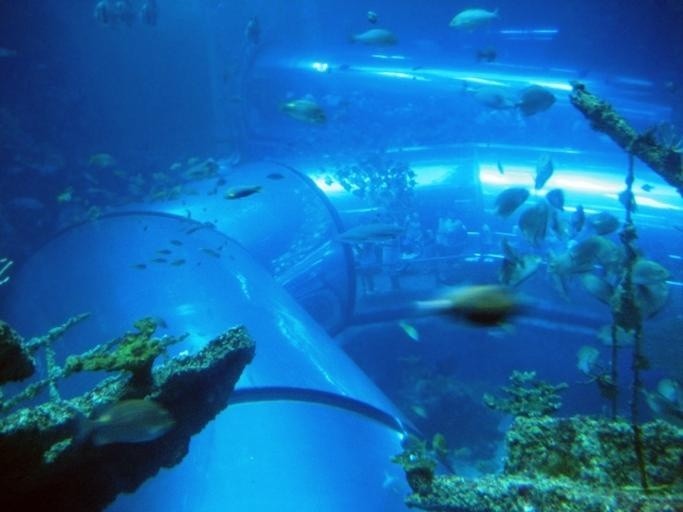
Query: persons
[359,208,493,295]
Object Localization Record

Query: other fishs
[266,5,683,511]
